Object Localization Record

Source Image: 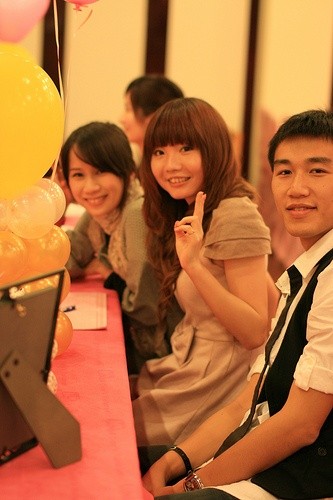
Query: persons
[44,73,333,500]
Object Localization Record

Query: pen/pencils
[62,306,76,312]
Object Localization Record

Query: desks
[1,274,154,500]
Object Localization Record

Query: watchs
[183,471,204,492]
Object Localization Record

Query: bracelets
[169,444,193,473]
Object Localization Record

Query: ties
[214,246,333,461]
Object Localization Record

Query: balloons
[0,0,73,392]
